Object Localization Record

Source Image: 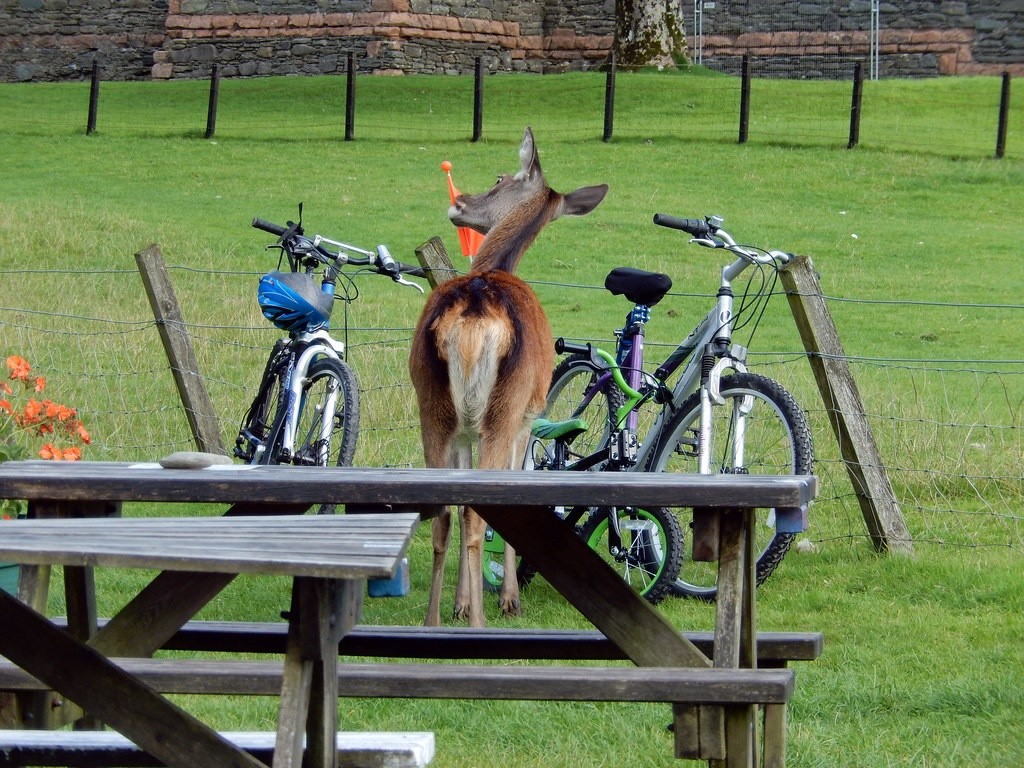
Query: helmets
[258,270,334,333]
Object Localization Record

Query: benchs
[47,616,822,768]
[1,655,795,768]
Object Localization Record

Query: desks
[0,513,419,768]
[1,453,816,768]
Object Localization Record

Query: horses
[407,124,608,630]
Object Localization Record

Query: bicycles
[520,213,822,604]
[476,337,685,610]
[232,201,432,518]
